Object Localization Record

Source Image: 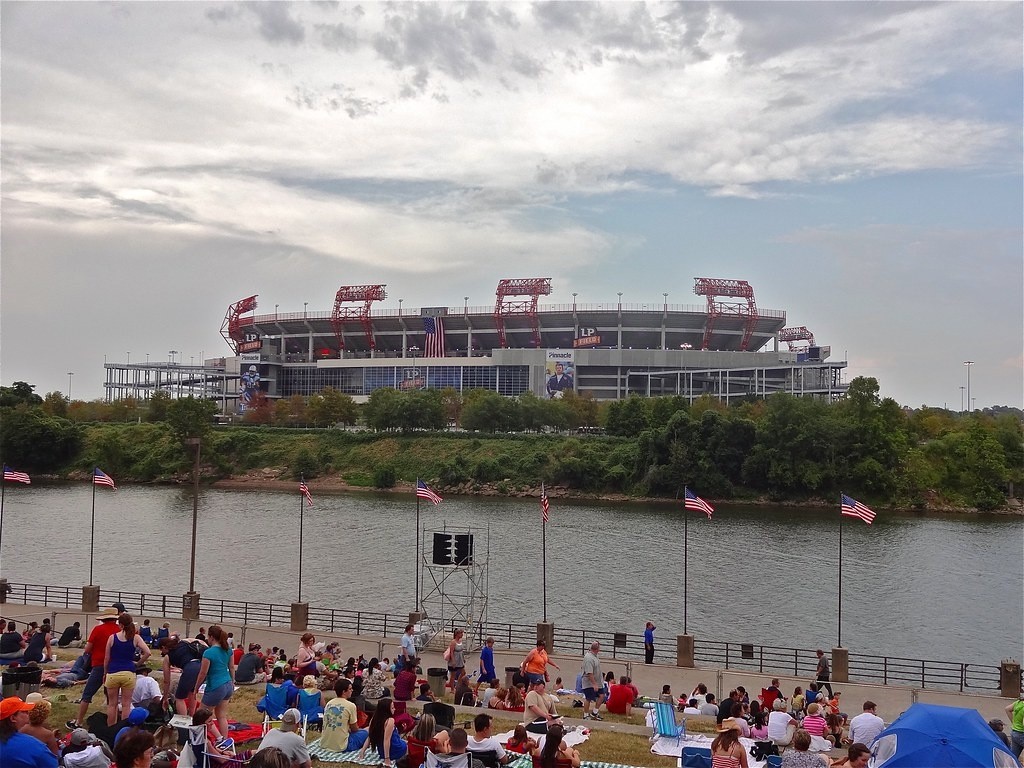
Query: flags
[541,485,550,522]
[93,466,114,491]
[423,317,445,357]
[417,477,443,505]
[841,493,877,525]
[686,487,716,520]
[300,478,313,507]
[4,466,31,484]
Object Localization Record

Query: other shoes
[215,738,225,747]
[645,661,655,664]
[843,723,851,726]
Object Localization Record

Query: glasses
[779,697,783,704]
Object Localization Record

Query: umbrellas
[867,701,1023,768]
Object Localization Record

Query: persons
[505,622,656,768]
[103,613,151,727]
[113,707,155,768]
[138,619,155,644]
[58,622,82,648]
[234,625,508,768]
[193,625,236,747]
[659,678,1024,768]
[0,692,116,768]
[154,708,312,768]
[816,649,832,696]
[65,602,128,732]
[158,638,202,716]
[240,365,260,402]
[547,363,573,400]
[155,622,171,645]
[0,618,57,661]
[131,664,164,710]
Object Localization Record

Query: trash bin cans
[505,667,520,689]
[427,668,448,697]
[2,667,43,701]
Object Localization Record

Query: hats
[0,697,35,721]
[816,693,823,701]
[95,608,120,620]
[716,718,742,735]
[112,603,128,613]
[25,692,51,709]
[280,708,301,732]
[128,706,150,726]
[70,728,89,746]
[806,703,823,717]
[834,692,841,695]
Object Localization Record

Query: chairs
[176,680,501,768]
[648,701,686,747]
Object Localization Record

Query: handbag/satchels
[152,724,179,752]
[544,670,550,682]
[443,647,451,662]
[179,638,209,654]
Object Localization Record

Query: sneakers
[590,711,602,721]
[65,720,82,731]
[583,714,589,721]
